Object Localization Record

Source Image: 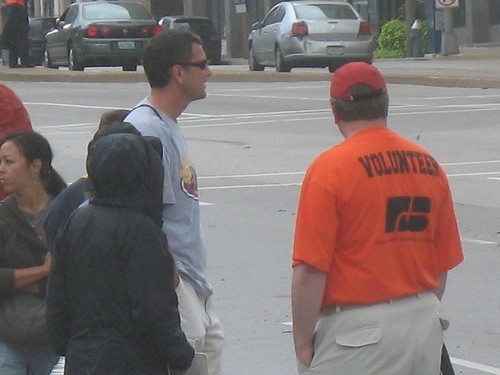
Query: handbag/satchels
[0,292,53,353]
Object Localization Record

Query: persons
[0,0,228,375]
[291,62,464,375]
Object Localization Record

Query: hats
[330,62,387,101]
[87,122,163,161]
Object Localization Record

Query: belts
[320,304,371,317]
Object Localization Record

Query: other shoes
[10,64,34,68]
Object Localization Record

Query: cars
[0,14,60,68]
[157,14,222,63]
[40,0,164,72]
[247,1,373,73]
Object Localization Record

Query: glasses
[170,59,207,70]
